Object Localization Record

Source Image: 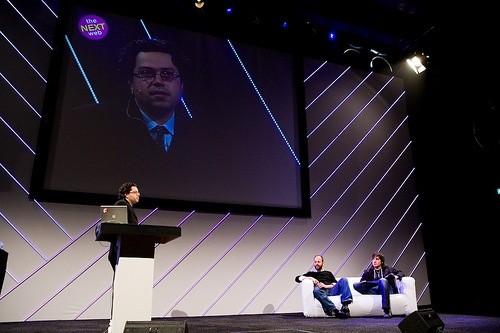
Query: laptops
[100,206,128,224]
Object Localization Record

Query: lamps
[406,50,431,74]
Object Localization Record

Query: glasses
[129,191,139,193]
[132,69,182,81]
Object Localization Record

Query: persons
[71,39,207,201]
[108,183,140,269]
[353,251,404,318]
[295,254,353,318]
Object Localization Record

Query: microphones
[132,87,141,92]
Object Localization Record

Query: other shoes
[340,306,350,317]
[384,311,392,318]
[335,312,346,318]
[386,273,394,280]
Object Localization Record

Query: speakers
[398,308,445,333]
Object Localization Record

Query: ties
[152,125,169,154]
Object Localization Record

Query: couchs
[301,277,418,318]
